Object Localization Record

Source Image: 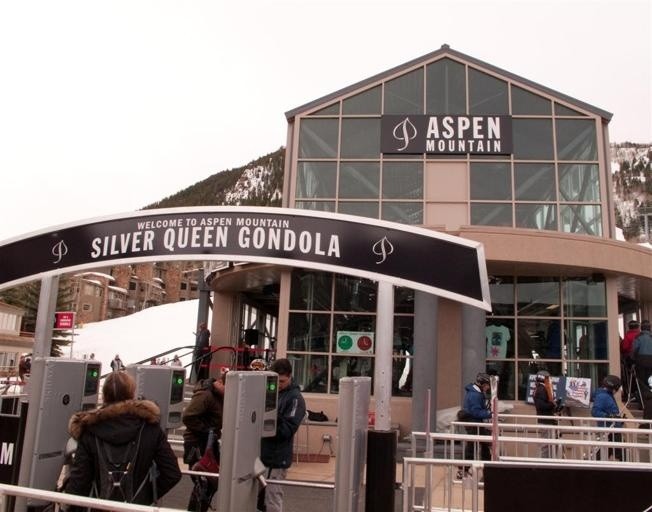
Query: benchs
[296,419,400,463]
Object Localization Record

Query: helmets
[536,370,550,379]
[603,375,621,387]
[477,373,490,383]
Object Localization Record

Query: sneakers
[457,471,471,481]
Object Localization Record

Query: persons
[590,375,627,460]
[55,372,183,512]
[19,356,31,379]
[621,320,652,437]
[456,373,494,479]
[110,354,125,372]
[183,357,306,512]
[150,354,183,367]
[194,322,210,375]
[532,371,565,457]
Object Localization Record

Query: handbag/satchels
[457,410,490,446]
[189,430,219,492]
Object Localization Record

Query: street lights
[67,269,169,363]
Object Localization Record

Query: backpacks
[93,430,143,503]
